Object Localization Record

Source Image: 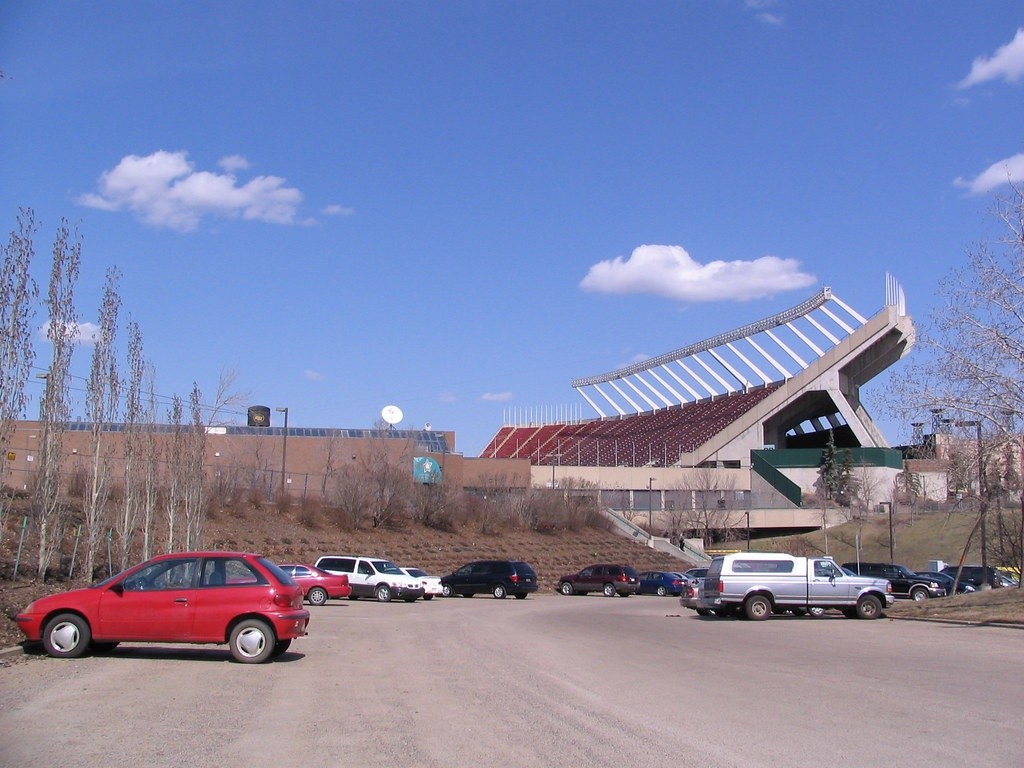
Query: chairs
[209,571,222,585]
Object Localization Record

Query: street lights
[275,406,289,499]
[954,420,988,585]
[34,373,50,412]
[547,460,555,489]
[434,431,446,496]
[744,512,749,551]
[880,501,893,563]
[649,476,656,538]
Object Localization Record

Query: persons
[679,537,684,551]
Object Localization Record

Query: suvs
[684,568,709,583]
[842,561,947,601]
[314,554,426,602]
[440,559,539,600]
[553,563,640,597]
[939,565,1002,589]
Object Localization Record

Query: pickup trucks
[693,550,895,620]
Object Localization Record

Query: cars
[654,572,699,587]
[912,570,976,596]
[385,566,444,600]
[13,550,311,664]
[1000,575,1018,588]
[226,562,352,606]
[638,570,689,597]
